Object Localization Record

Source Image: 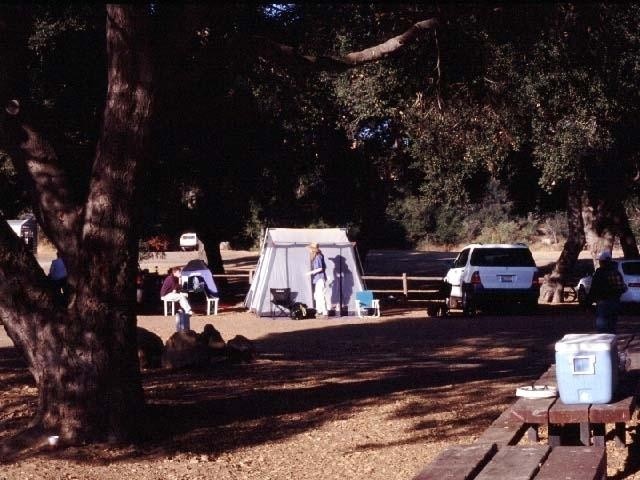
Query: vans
[180,231,199,251]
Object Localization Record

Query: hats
[306,242,321,253]
[596,253,609,260]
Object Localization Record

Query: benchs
[412,333,640,480]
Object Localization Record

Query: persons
[48,251,67,293]
[197,323,226,353]
[158,266,194,316]
[305,240,329,319]
[584,253,627,334]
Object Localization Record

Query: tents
[244,226,372,318]
[178,258,222,295]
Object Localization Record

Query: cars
[576,257,640,306]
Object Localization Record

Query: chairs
[355,290,380,318]
[270,288,297,318]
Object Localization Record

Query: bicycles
[562,284,577,302]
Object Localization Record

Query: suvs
[443,242,541,314]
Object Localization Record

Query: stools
[163,300,176,317]
